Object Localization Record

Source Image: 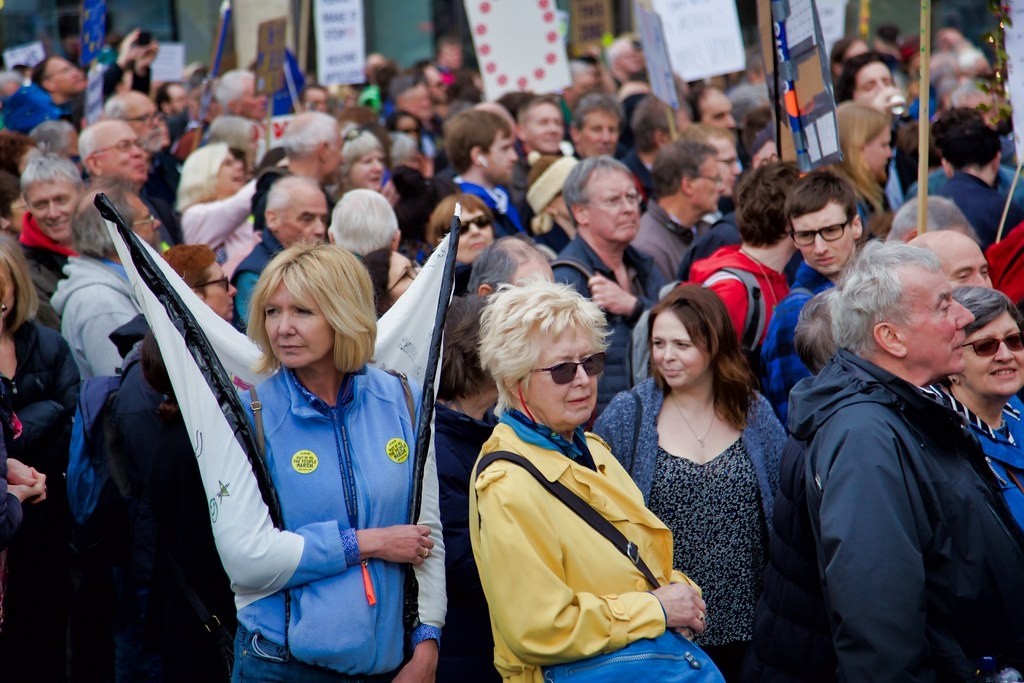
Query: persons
[0,23,1024,683]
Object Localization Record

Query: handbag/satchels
[541,628,726,683]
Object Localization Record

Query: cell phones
[136,31,152,46]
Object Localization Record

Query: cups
[890,95,907,115]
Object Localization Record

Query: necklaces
[671,392,716,446]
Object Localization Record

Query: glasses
[442,215,490,237]
[529,352,605,384]
[191,276,230,293]
[94,140,144,154]
[128,111,162,124]
[791,220,851,246]
[698,176,723,187]
[964,331,1024,357]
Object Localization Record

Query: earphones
[478,154,488,168]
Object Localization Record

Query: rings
[687,628,692,636]
[699,609,705,622]
[422,548,428,558]
